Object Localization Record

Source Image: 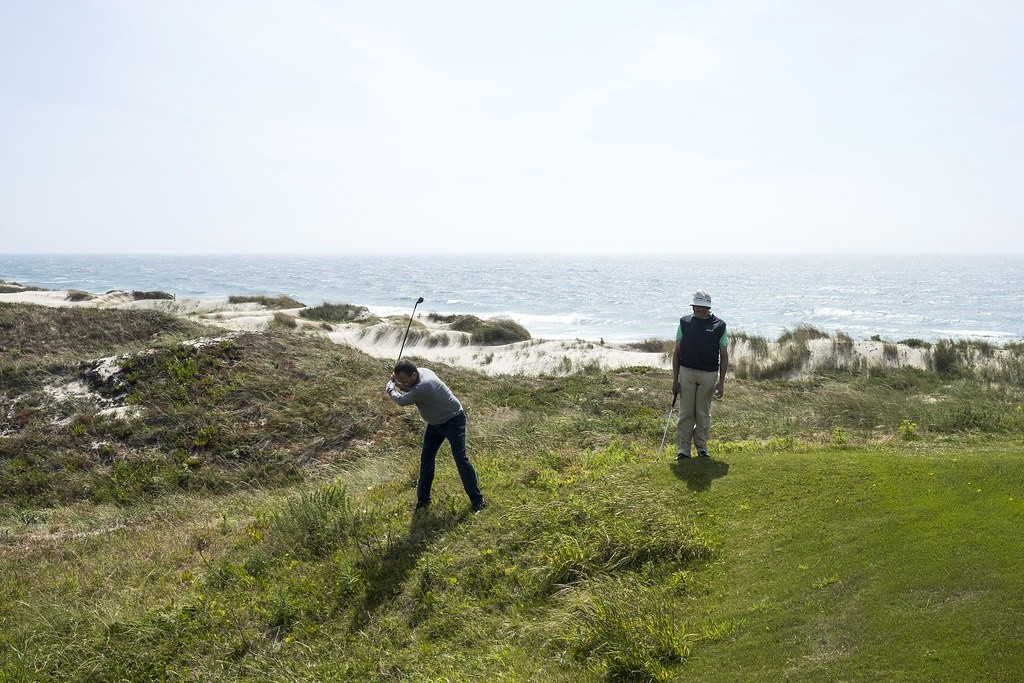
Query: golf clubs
[396,296,424,363]
[653,391,678,466]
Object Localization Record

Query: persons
[671,288,729,462]
[386,359,490,514]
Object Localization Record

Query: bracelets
[388,388,395,397]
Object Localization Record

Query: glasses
[396,376,410,386]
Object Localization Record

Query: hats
[690,290,712,309]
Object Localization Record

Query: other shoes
[472,503,485,512]
[674,453,689,462]
[698,451,711,457]
